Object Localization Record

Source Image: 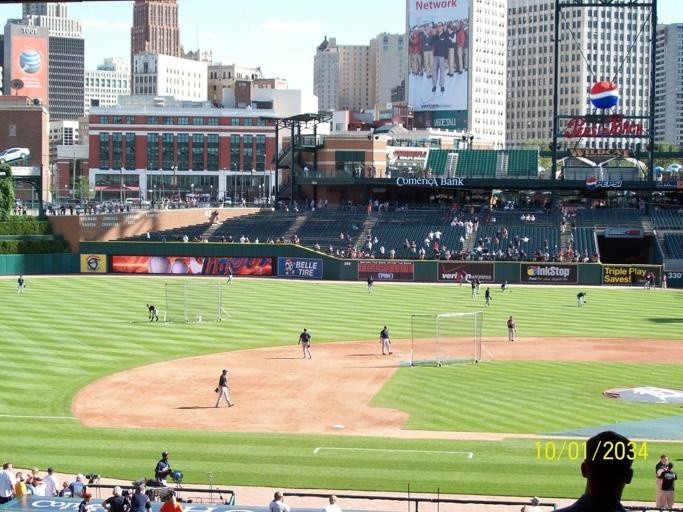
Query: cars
[0,146,30,163]
[44,202,62,212]
[65,197,119,214]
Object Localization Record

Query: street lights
[117,152,268,210]
[461,133,474,178]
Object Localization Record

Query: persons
[409,18,469,93]
[577,293,586,306]
[458,270,507,306]
[643,272,655,287]
[657,463,678,512]
[367,275,373,291]
[268,491,289,512]
[225,266,233,284]
[506,316,516,342]
[656,454,669,507]
[662,275,671,288]
[147,304,159,322]
[551,430,635,512]
[0,451,184,511]
[11,163,683,262]
[379,325,393,355]
[214,369,234,407]
[520,496,542,512]
[297,328,312,359]
[322,495,341,512]
[16,274,26,294]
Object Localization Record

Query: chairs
[416,143,541,179]
[106,207,682,265]
